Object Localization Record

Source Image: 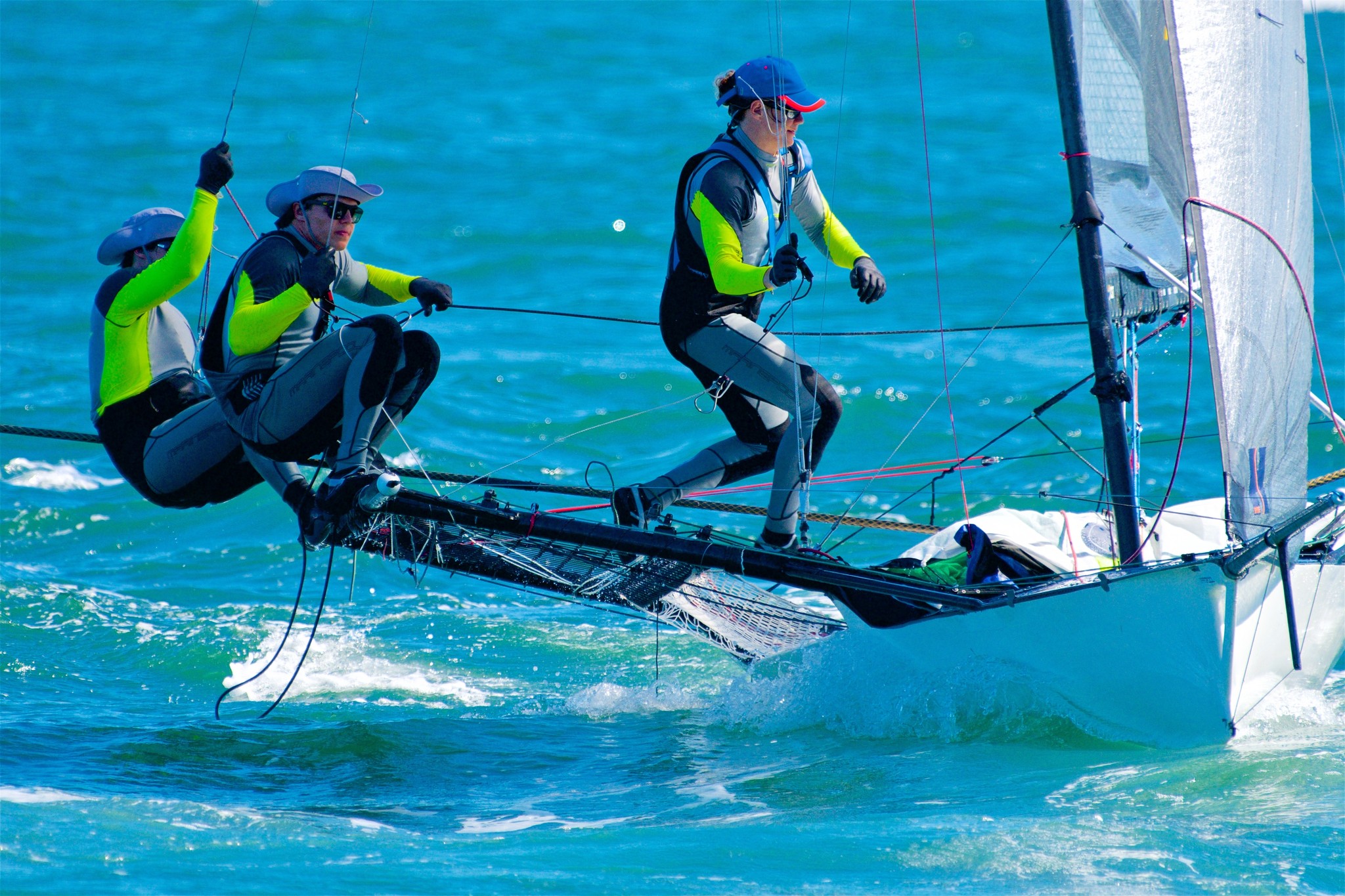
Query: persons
[200,166,455,517]
[87,141,387,528]
[609,56,886,566]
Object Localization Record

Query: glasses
[766,98,801,119]
[303,200,363,224]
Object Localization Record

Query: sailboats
[215,0,1343,751]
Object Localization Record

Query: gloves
[298,245,336,299]
[410,277,455,319]
[849,257,886,305]
[194,140,233,196]
[770,232,800,287]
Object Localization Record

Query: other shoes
[295,507,338,546]
[320,467,367,495]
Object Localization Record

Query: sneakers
[754,534,802,555]
[610,481,651,568]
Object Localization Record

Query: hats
[716,57,826,113]
[96,208,218,267]
[266,168,382,217]
